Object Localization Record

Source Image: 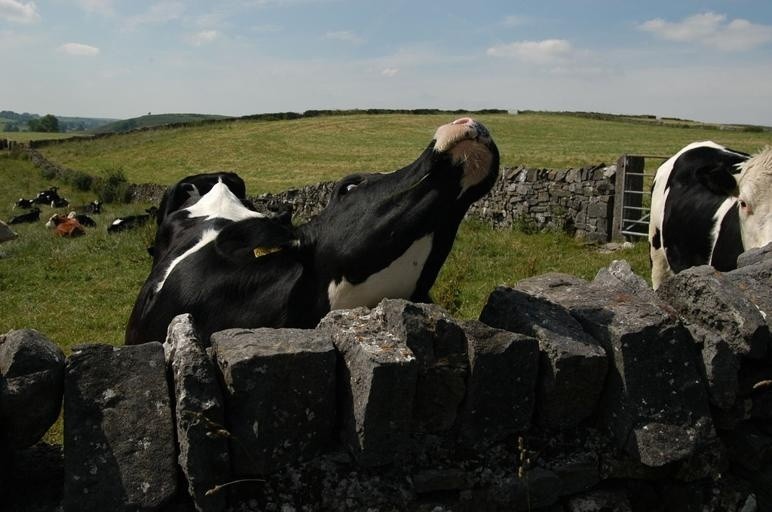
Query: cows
[646,139,772,293]
[106,205,159,237]
[6,184,104,238]
[125,115,500,345]
